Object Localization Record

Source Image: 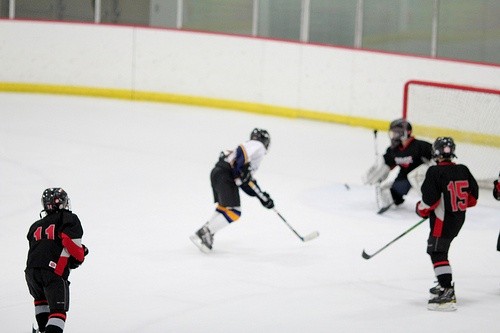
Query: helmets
[42,188,68,214]
[250,128,270,150]
[388,120,412,149]
[431,136,455,158]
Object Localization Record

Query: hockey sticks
[246,180,319,243]
[362,214,430,260]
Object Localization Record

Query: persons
[24,187,89,333]
[493,174,500,253]
[192,128,273,252]
[414,136,479,311]
[370,119,439,212]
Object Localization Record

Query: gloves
[257,192,274,209]
[416,201,431,220]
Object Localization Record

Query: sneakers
[189,226,214,253]
[429,281,446,300]
[427,283,458,311]
